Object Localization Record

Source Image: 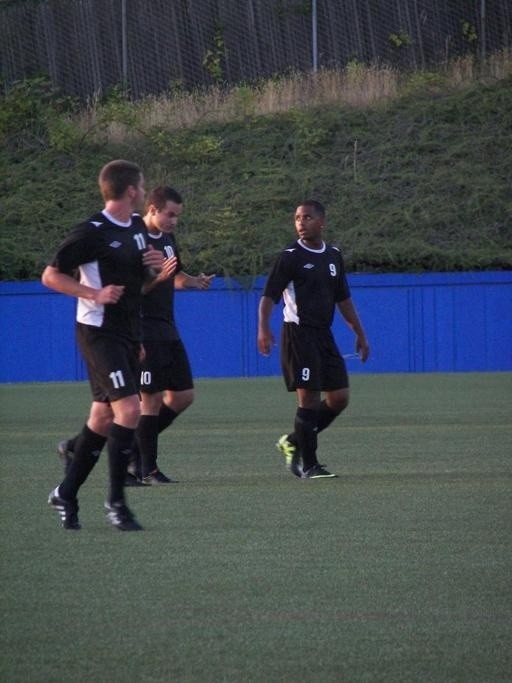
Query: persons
[258,201,370,479]
[127,186,210,488]
[41,156,164,531]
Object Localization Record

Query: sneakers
[47,484,81,530]
[141,468,180,486]
[57,439,74,474]
[275,433,302,478]
[123,472,152,487]
[103,498,144,532]
[301,464,336,479]
